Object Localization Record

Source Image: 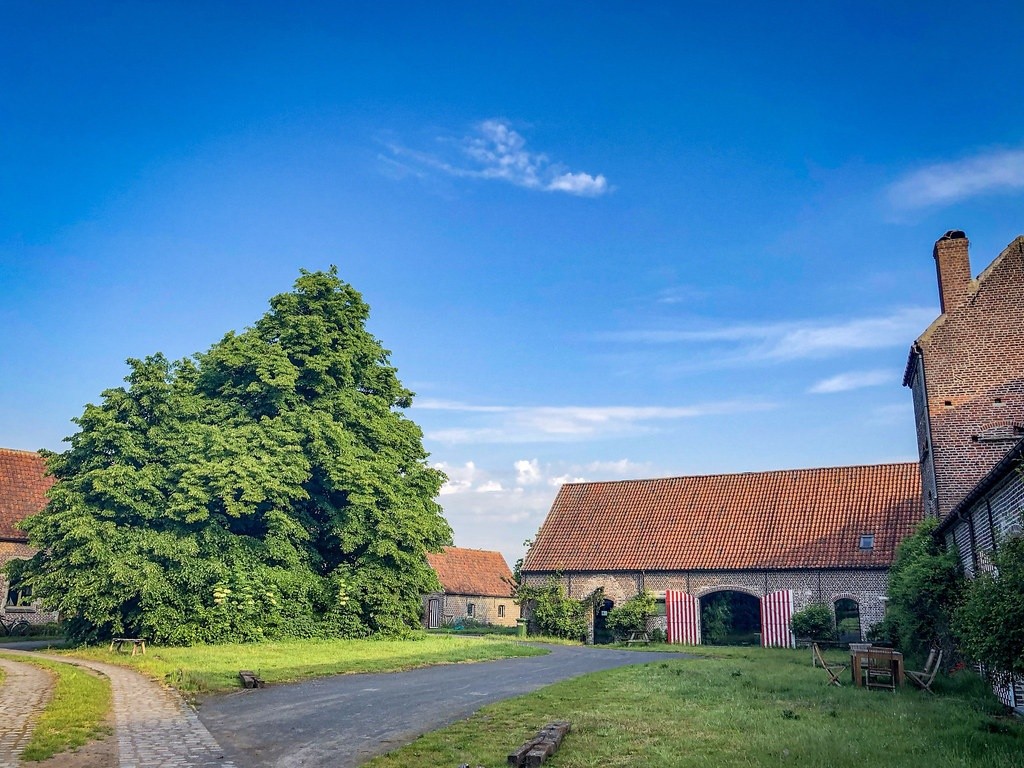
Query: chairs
[867,647,895,693]
[813,643,851,687]
[904,649,944,694]
[849,643,877,682]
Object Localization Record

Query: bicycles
[0,615,34,638]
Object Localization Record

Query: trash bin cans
[516,618,530,637]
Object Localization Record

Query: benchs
[108,638,146,657]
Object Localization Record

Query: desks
[849,649,904,688]
[625,630,652,647]
